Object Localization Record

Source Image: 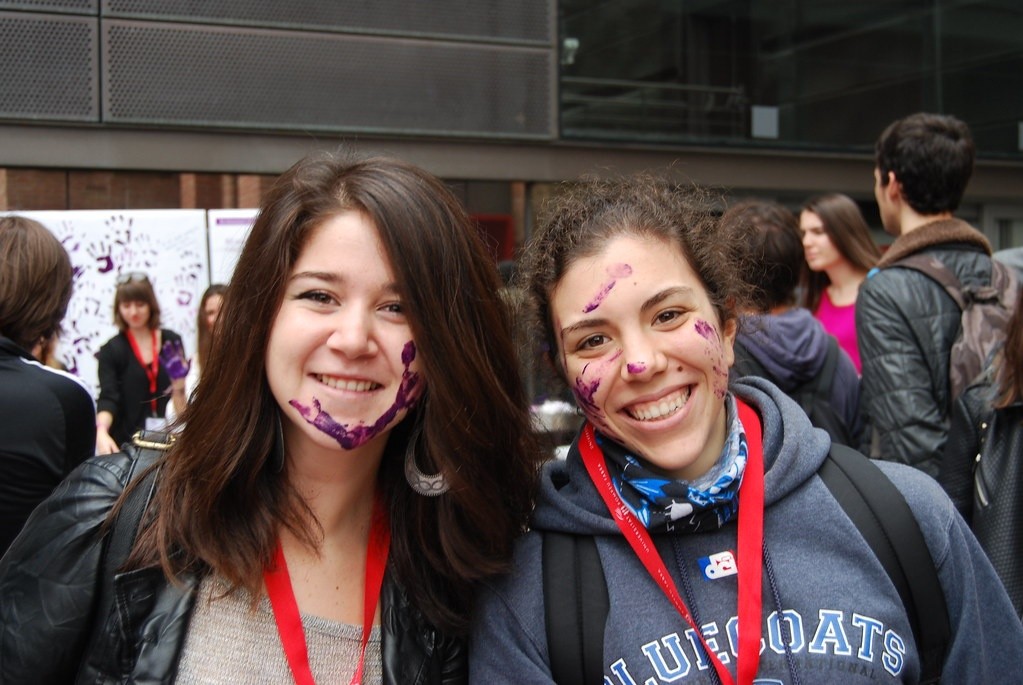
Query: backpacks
[882,252,1019,404]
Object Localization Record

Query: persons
[160,285,229,421]
[0,155,547,685]
[470,175,1023,685]
[0,216,97,560]
[855,115,1023,520]
[691,192,888,449]
[94,271,183,458]
[495,257,582,453]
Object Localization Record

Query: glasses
[116,272,147,284]
